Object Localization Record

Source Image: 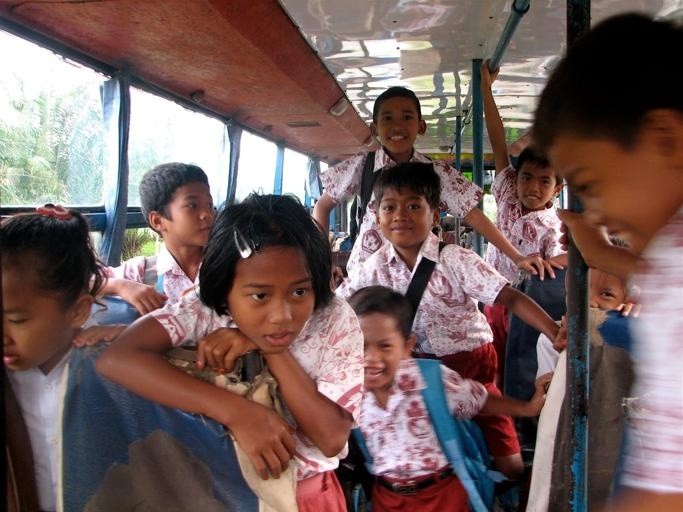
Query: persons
[1,162,569,512]
[311,14,683,510]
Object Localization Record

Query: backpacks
[352,359,510,512]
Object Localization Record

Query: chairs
[91,232,571,477]
